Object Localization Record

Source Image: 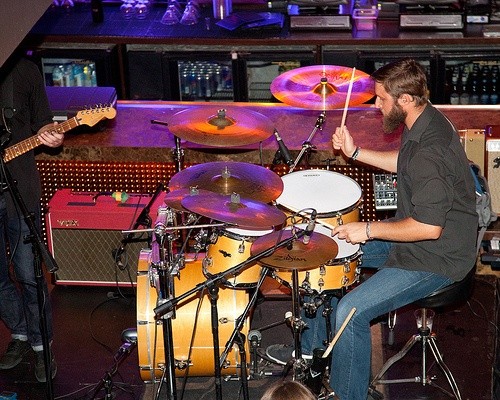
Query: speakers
[249,294,301,369]
[44,186,157,287]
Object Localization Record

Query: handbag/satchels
[469,163,497,227]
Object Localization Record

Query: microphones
[112,247,124,270]
[303,209,317,245]
[273,127,295,168]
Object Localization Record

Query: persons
[259,380,318,400]
[0,54,64,383]
[266,57,479,400]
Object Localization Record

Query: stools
[368,286,464,400]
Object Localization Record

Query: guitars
[0,102,117,192]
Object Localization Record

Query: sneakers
[0,339,31,369]
[34,349,58,383]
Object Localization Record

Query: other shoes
[266,344,297,364]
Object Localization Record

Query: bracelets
[366,221,374,240]
[352,146,360,160]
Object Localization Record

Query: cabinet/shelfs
[32,3,500,107]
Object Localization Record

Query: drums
[274,168,363,230]
[202,219,274,289]
[271,223,362,292]
[135,248,252,380]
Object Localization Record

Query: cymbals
[168,105,274,147]
[181,195,287,228]
[250,228,338,268]
[164,188,215,212]
[270,65,376,111]
[169,161,284,205]
